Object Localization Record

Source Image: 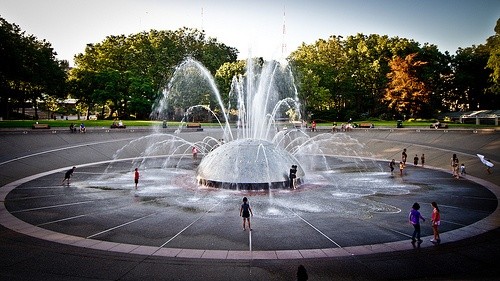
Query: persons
[311,121,317,132]
[414,154,419,165]
[389,158,396,175]
[450,154,460,178]
[486,159,493,174]
[399,161,404,175]
[290,164,297,190]
[63,166,77,184]
[429,202,442,242]
[239,197,253,231]
[331,121,355,133]
[135,167,141,188]
[434,121,440,130]
[460,164,466,174]
[421,153,425,167]
[70,123,86,133]
[409,202,425,245]
[192,144,198,162]
[402,148,408,167]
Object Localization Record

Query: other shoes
[430,239,437,242]
[417,239,423,243]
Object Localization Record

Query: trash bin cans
[397,120,401,128]
[163,120,167,128]
[333,121,337,126]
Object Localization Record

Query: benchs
[359,121,374,128]
[293,122,308,128]
[430,123,449,129]
[33,124,51,129]
[187,122,201,128]
[112,123,127,129]
